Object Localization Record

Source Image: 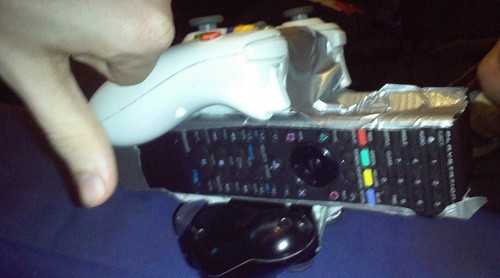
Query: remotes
[114,113,476,217]
